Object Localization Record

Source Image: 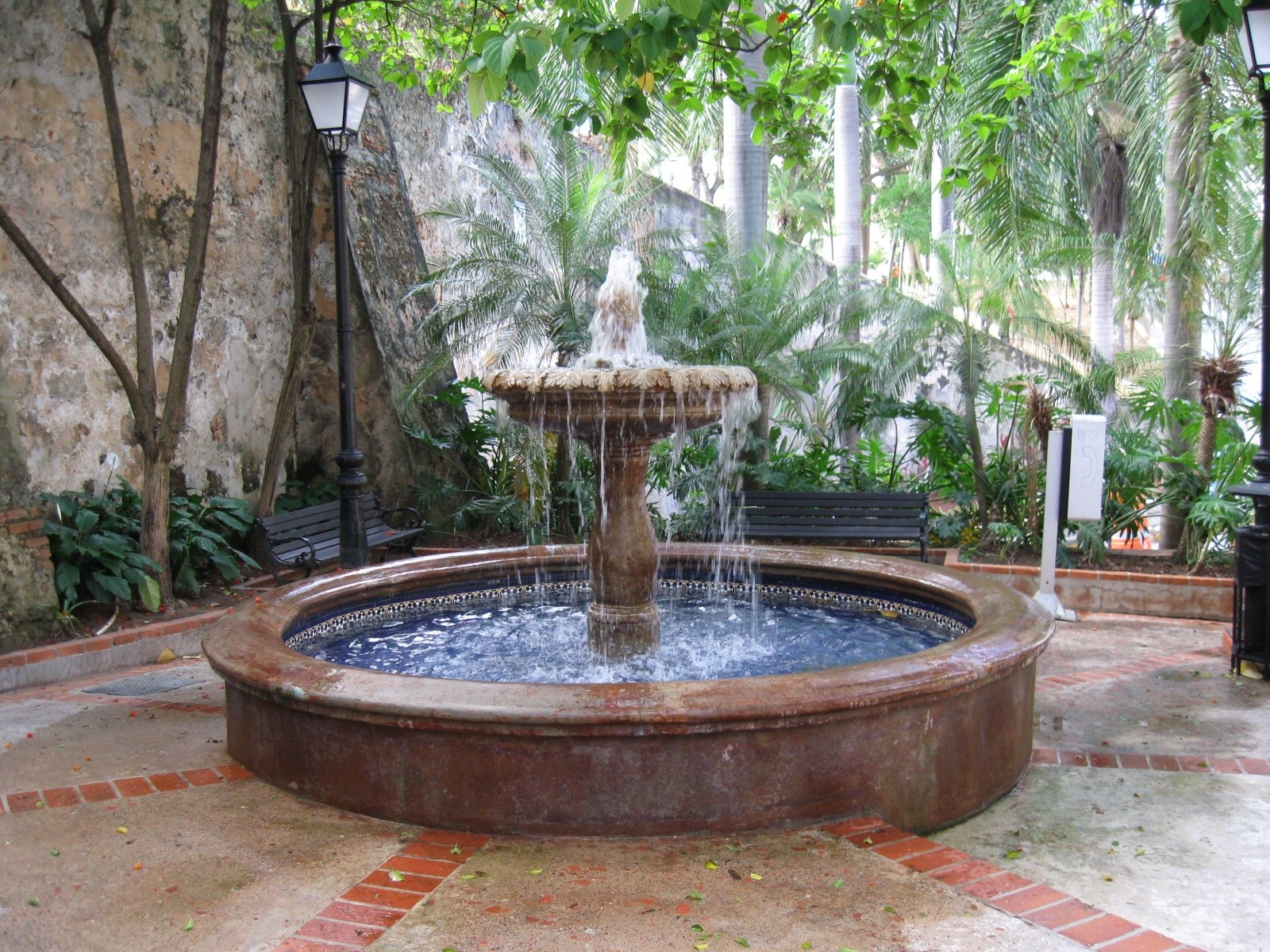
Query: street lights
[298,38,378,569]
[1226,0,1270,681]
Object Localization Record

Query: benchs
[254,489,424,586]
[710,489,932,563]
